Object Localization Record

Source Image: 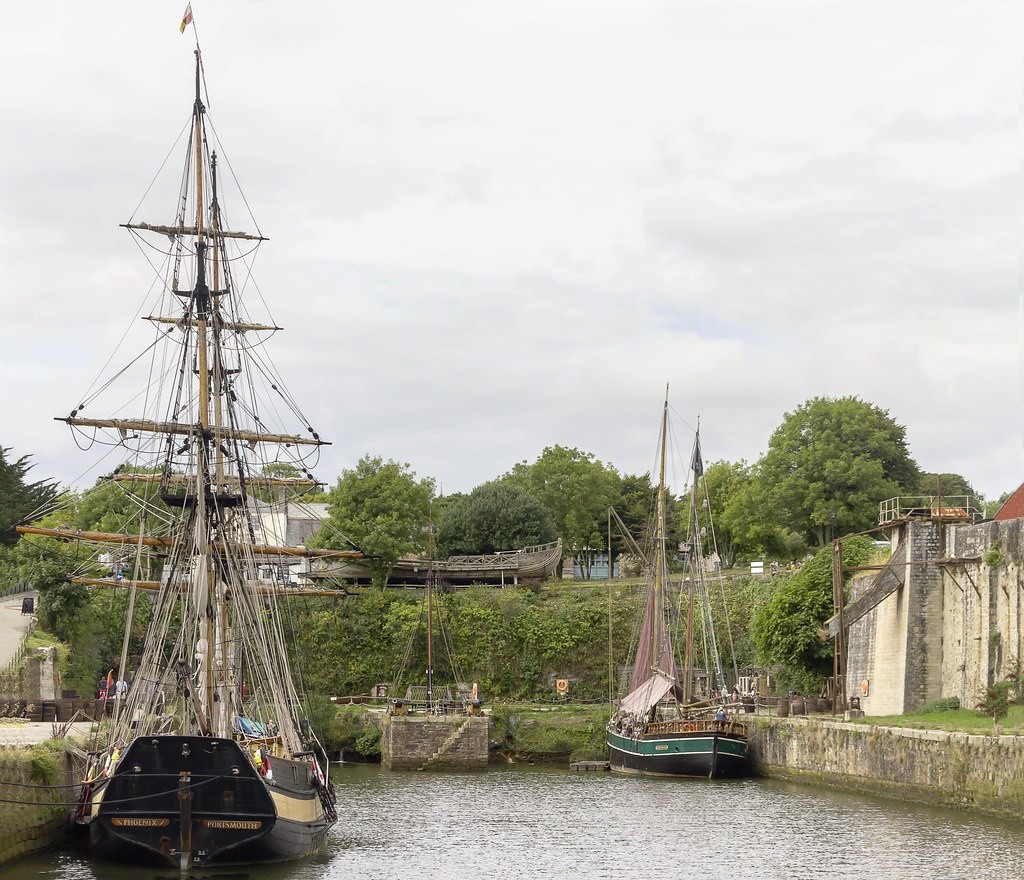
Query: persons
[616,716,623,734]
[94,673,106,699]
[116,676,128,699]
[716,707,727,720]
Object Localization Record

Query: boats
[297,537,563,590]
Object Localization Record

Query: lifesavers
[684,722,696,732]
[311,758,325,787]
[557,680,568,691]
[103,748,119,776]
[260,756,274,782]
[377,686,388,696]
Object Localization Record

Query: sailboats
[603,381,751,783]
[385,511,484,716]
[4,4,444,879]
